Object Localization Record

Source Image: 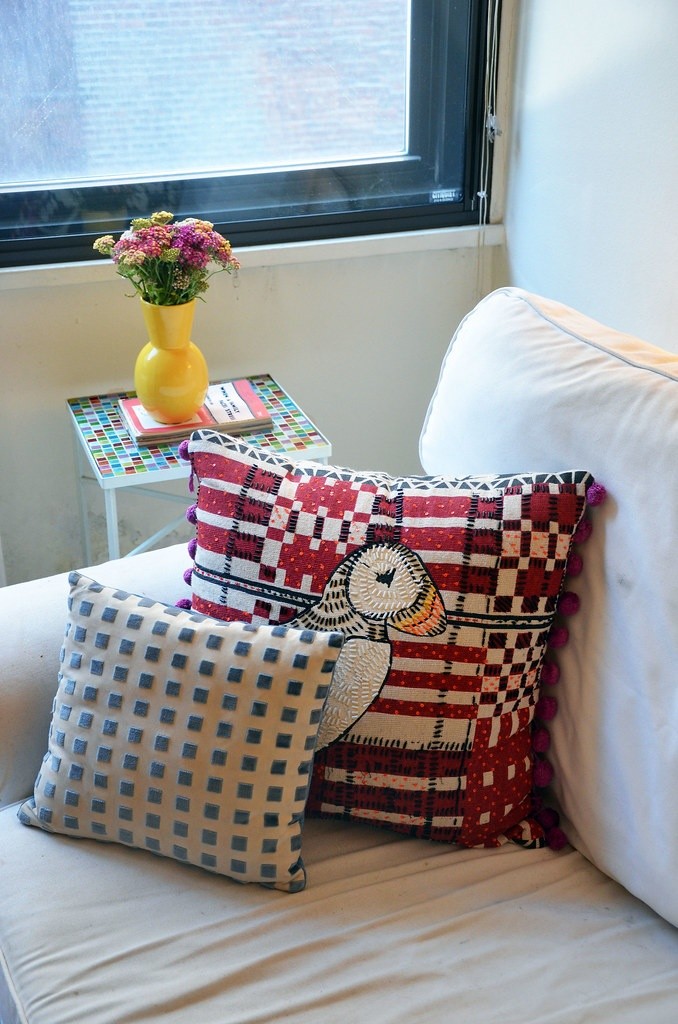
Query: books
[116,378,272,438]
[116,407,275,447]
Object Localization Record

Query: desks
[65,372,334,568]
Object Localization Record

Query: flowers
[92,209,241,306]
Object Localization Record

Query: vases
[134,296,210,424]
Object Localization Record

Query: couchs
[0,287,678,1024]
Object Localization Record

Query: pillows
[177,429,608,853]
[15,569,347,894]
